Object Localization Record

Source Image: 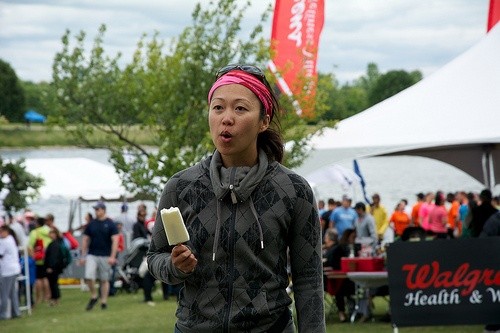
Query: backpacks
[57,244,71,267]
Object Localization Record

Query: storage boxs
[340,257,385,273]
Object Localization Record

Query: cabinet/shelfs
[15,245,32,316]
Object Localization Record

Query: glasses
[215,64,266,85]
[95,208,99,210]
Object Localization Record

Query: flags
[266,0,325,117]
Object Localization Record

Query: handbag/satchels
[33,230,45,259]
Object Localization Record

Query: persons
[0,210,72,319]
[319,188,500,320]
[147,67,326,333]
[79,203,156,310]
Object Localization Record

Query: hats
[25,212,34,218]
[343,194,351,200]
[93,203,105,208]
[352,202,365,210]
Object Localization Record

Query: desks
[347,272,389,326]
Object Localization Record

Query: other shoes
[339,311,345,320]
[86,297,98,311]
[101,303,107,309]
[358,315,372,323]
[147,301,155,306]
